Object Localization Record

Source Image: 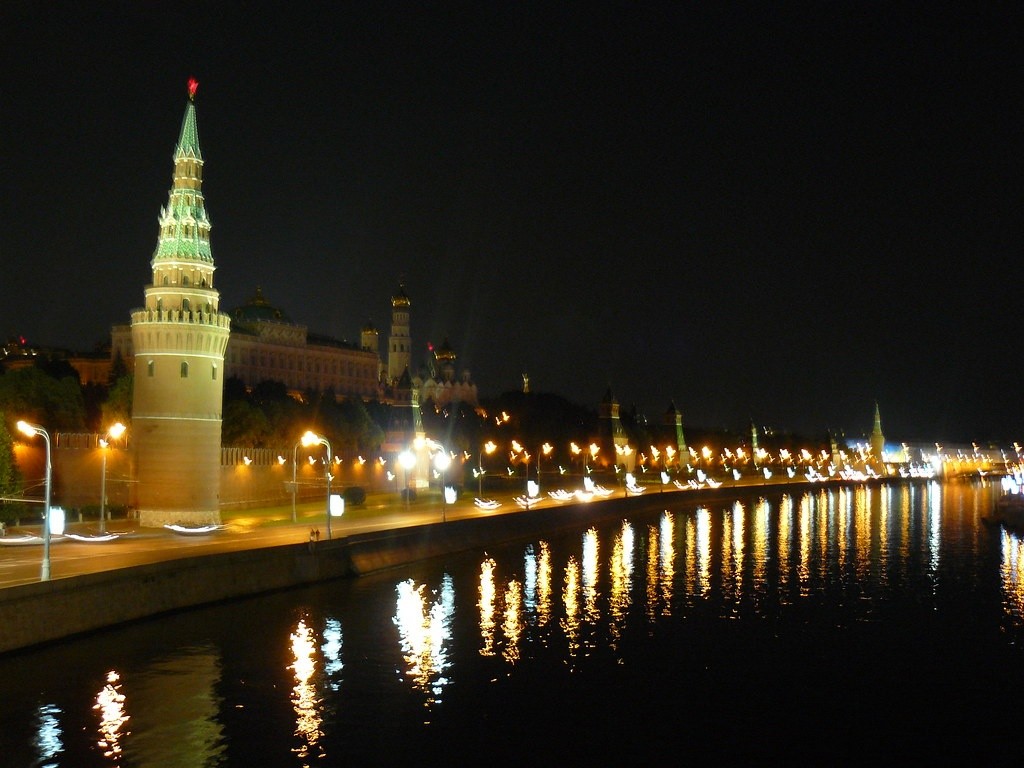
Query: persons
[311,528,320,541]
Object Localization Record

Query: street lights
[397,449,412,508]
[99,424,128,536]
[289,432,308,523]
[512,441,529,501]
[569,441,1023,502]
[413,438,448,523]
[309,434,331,539]
[479,441,497,502]
[16,420,51,582]
[537,442,555,498]
